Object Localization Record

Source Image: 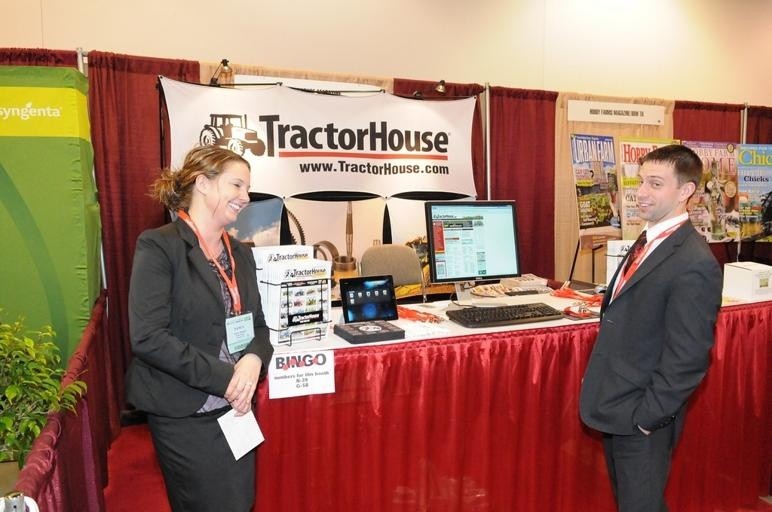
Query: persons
[579,144,724,512]
[125,146,275,512]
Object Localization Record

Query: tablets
[339,275,399,323]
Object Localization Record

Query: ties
[623,231,646,273]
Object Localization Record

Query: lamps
[211,56,230,85]
[413,79,446,98]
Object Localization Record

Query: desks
[254,290,772,512]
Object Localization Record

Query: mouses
[564,306,591,317]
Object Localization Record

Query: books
[573,134,772,250]
[500,273,548,288]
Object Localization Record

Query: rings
[245,383,252,388]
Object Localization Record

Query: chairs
[357,244,428,304]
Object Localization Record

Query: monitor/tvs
[424,199,521,306]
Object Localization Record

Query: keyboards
[446,302,565,327]
